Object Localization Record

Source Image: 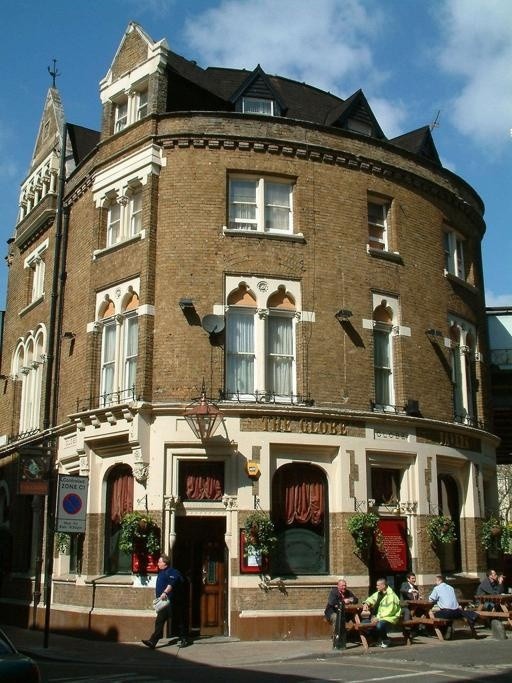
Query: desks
[340,591,512,652]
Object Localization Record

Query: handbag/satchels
[152,595,171,611]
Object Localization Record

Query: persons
[362,578,402,648]
[142,553,189,650]
[428,576,462,636]
[475,568,505,612]
[399,572,427,636]
[324,580,358,632]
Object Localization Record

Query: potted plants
[480,512,512,556]
[346,512,388,561]
[119,510,161,555]
[426,512,457,546]
[243,508,280,557]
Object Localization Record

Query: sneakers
[141,639,156,650]
[179,637,191,648]
[377,639,391,648]
[445,626,456,639]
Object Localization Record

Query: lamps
[64,331,77,338]
[179,299,193,306]
[335,309,352,318]
[425,328,442,337]
[181,375,224,444]
[0,374,8,380]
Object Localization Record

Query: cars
[0,628,42,683]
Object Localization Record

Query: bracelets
[163,592,168,595]
